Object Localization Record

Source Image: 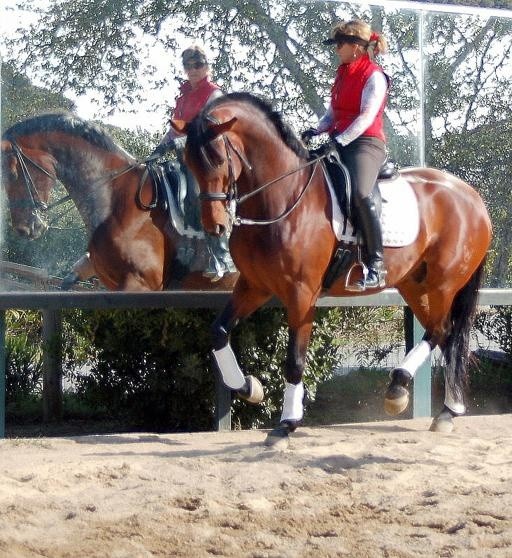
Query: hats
[322,32,367,46]
[183,54,204,64]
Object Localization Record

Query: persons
[307,19,393,291]
[151,46,237,276]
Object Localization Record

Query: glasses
[184,62,204,70]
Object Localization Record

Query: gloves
[301,127,322,146]
[317,142,336,155]
[156,140,175,154]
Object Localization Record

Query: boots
[352,192,386,288]
[202,233,237,278]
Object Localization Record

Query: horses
[0,111,239,294]
[169,90,492,448]
